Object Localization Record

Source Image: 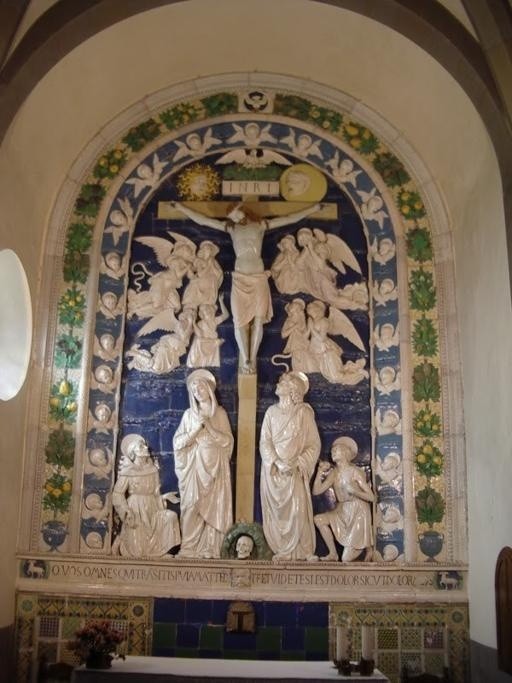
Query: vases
[86,648,112,668]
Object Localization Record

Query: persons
[126,240,230,374]
[312,443,375,562]
[172,375,234,559]
[260,374,321,561]
[166,200,331,374]
[272,228,369,384]
[112,440,181,557]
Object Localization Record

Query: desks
[73,653,389,683]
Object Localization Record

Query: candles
[361,625,374,660]
[336,625,348,661]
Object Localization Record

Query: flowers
[65,618,126,663]
